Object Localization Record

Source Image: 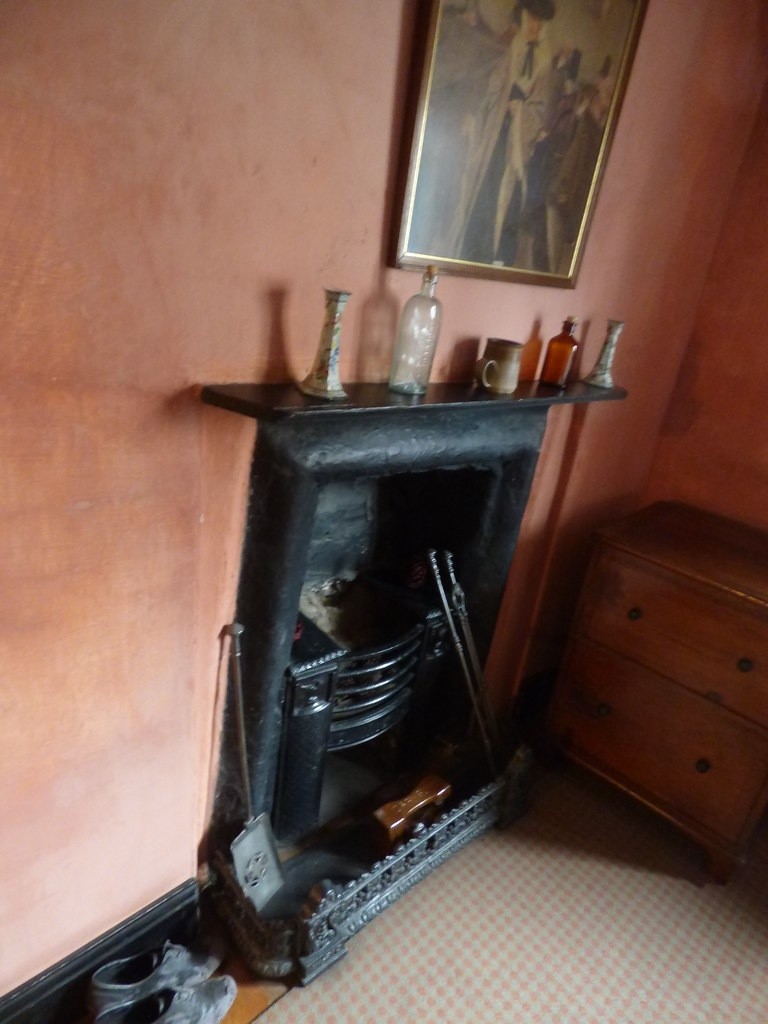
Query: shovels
[222,622,286,914]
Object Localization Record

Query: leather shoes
[85,931,235,1024]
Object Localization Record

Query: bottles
[540,315,579,389]
[388,265,443,396]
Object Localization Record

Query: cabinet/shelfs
[522,499,768,861]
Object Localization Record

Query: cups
[479,337,524,394]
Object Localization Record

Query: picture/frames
[387,0,650,289]
